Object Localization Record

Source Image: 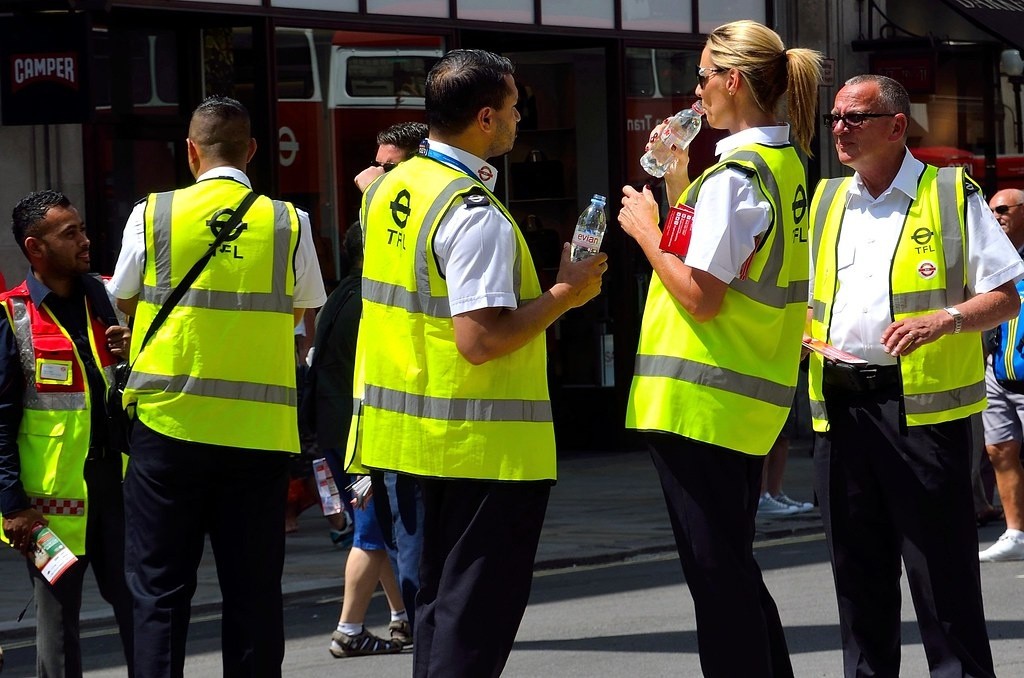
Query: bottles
[570,194,607,265]
[640,100,706,179]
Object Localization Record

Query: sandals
[388,619,414,648]
[330,624,403,658]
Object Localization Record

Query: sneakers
[775,492,814,513]
[757,492,799,514]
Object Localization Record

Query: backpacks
[989,278,1024,395]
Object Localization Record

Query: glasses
[991,203,1023,214]
[823,113,895,128]
[697,67,759,90]
[371,160,398,173]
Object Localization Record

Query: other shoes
[331,512,359,547]
[978,533,1024,561]
[976,506,1005,523]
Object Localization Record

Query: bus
[0,0,729,456]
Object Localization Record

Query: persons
[617,18,823,677]
[979,189,1024,563]
[345,50,607,678]
[286,476,354,549]
[801,75,1024,678]
[353,122,430,191]
[756,398,814,515]
[0,192,132,678]
[106,100,327,678]
[315,219,413,659]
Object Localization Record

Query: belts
[823,361,902,390]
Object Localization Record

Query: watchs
[944,305,962,335]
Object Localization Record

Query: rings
[908,334,914,339]
